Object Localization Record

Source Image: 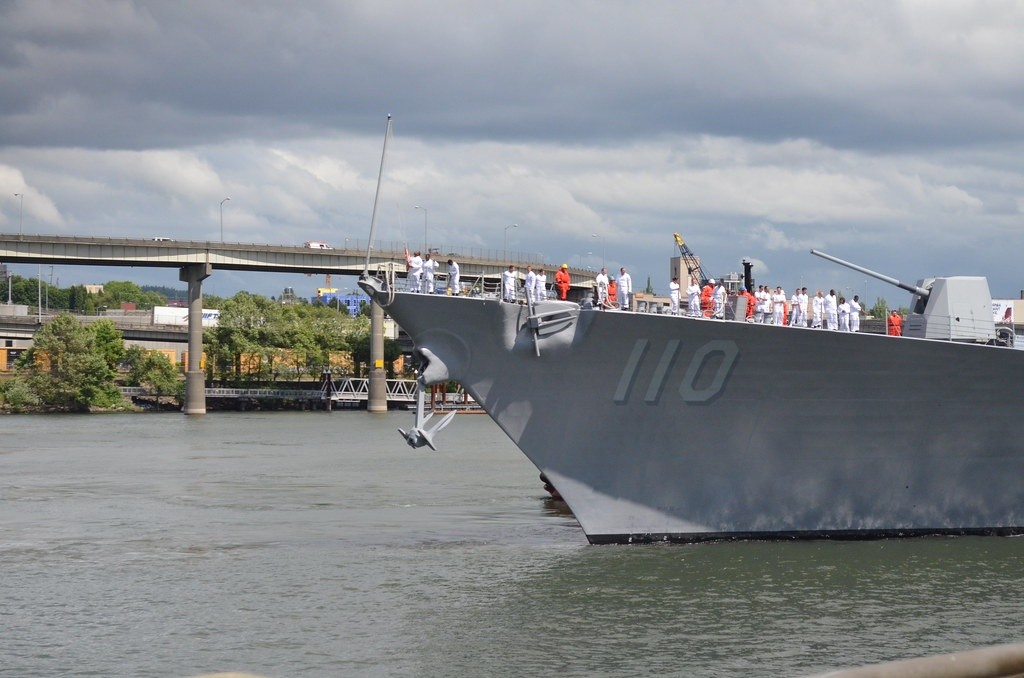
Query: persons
[737,285,788,325]
[669,277,680,315]
[536,269,547,300]
[504,266,516,302]
[789,287,808,327]
[700,279,716,319]
[686,278,701,317]
[709,281,726,319]
[554,263,570,300]
[524,266,536,303]
[423,255,439,293]
[812,289,868,332]
[447,259,460,295]
[407,251,422,293]
[596,268,632,310]
[888,309,901,336]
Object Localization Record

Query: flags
[404,248,413,271]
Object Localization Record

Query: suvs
[153,238,172,241]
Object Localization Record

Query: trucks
[305,241,332,249]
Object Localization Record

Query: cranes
[304,273,342,295]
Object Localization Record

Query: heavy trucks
[151,306,219,327]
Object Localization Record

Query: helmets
[609,276,615,280]
[561,264,568,269]
[739,286,745,291]
[708,279,716,284]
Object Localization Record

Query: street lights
[581,253,592,266]
[414,205,428,253]
[97,306,107,316]
[220,197,231,244]
[504,224,519,261]
[591,234,605,268]
[15,193,24,240]
[345,238,349,250]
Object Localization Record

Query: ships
[357,110,1024,546]
